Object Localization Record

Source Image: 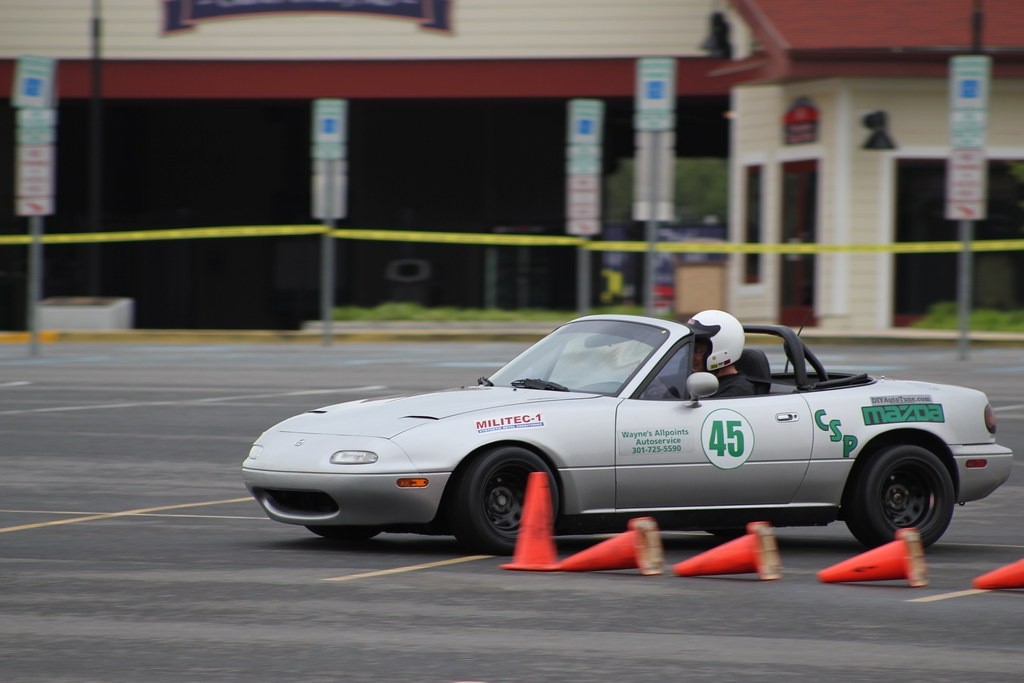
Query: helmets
[687,310,745,371]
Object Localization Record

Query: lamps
[863,111,897,150]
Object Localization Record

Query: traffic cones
[971,558,1024,589]
[551,517,669,577]
[820,527,931,587]
[664,522,782,582]
[498,470,563,572]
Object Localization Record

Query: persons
[644,310,755,400]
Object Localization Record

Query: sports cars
[246,313,1013,556]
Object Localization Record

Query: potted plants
[28,295,135,331]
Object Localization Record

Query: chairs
[737,348,772,395]
